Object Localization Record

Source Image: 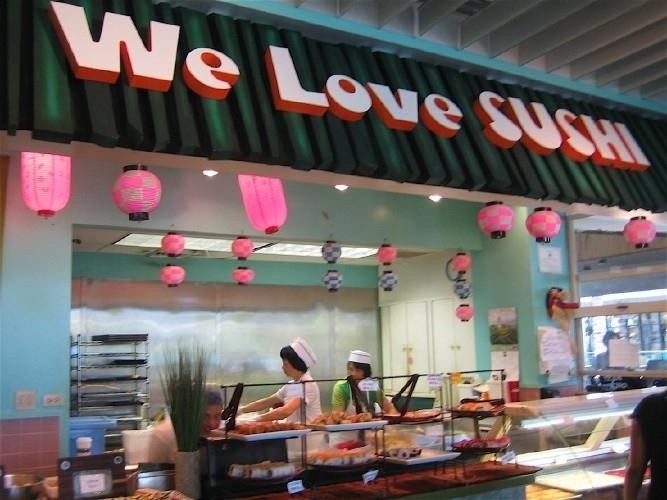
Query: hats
[348,350,371,364]
[291,337,317,368]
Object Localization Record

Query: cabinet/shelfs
[67,331,151,447]
[209,365,537,500]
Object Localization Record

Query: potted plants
[155,337,216,500]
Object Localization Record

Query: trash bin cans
[138,462,176,491]
[68,415,117,457]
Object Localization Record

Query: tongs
[220,382,244,431]
[391,374,419,417]
[460,398,506,406]
[347,375,375,415]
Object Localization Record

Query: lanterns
[377,245,398,291]
[160,232,184,286]
[238,174,288,234]
[624,217,656,248]
[454,253,474,321]
[321,241,343,293]
[478,202,515,239]
[232,237,254,284]
[526,208,561,243]
[21,151,71,216]
[112,165,162,221]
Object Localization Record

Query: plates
[372,448,461,466]
[228,429,312,441]
[225,464,305,485]
[451,437,512,452]
[382,411,441,422]
[306,420,388,432]
[304,455,379,472]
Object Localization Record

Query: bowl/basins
[43,476,59,499]
[0,473,44,500]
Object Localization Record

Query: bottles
[76,437,93,457]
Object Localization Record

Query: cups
[508,381,520,402]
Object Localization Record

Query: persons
[199,391,223,431]
[236,338,321,424]
[332,350,398,419]
[624,389,666,500]
[596,331,624,369]
